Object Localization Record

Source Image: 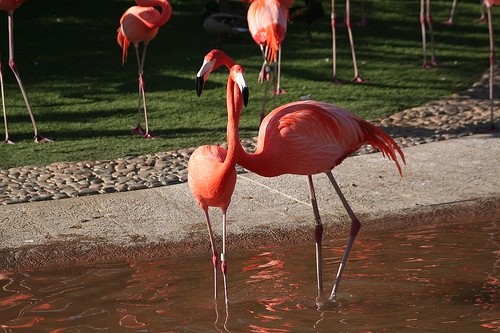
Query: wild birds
[329,0,368,86]
[478,0,500,133]
[247,0,289,96]
[194,50,407,307]
[419,0,446,70]
[441,0,488,27]
[0,0,55,147]
[188,65,250,305]
[117,0,173,142]
